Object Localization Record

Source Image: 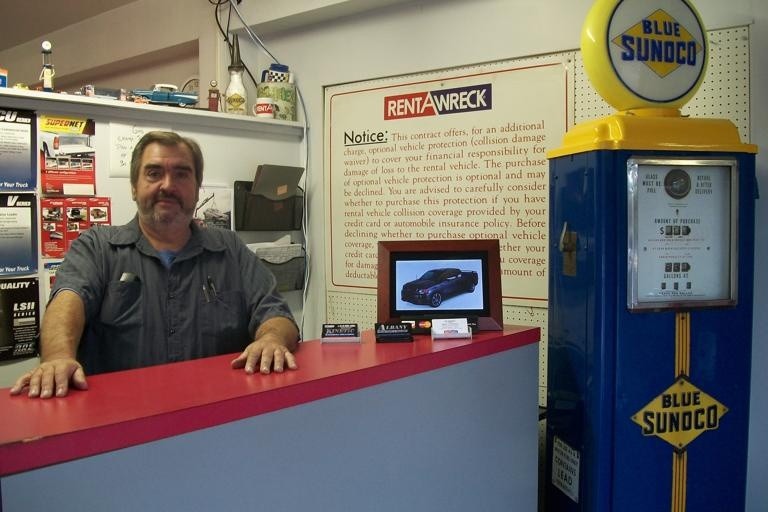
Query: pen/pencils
[202,276,217,303]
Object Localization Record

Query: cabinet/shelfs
[232,179,308,293]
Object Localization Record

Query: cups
[252,97,274,119]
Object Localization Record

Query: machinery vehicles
[193,193,214,227]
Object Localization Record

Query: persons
[9,130,301,400]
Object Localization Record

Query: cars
[91,209,105,218]
[401,268,478,307]
[67,208,81,221]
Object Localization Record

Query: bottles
[224,33,248,115]
[261,61,289,83]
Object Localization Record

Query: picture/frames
[376,238,504,333]
